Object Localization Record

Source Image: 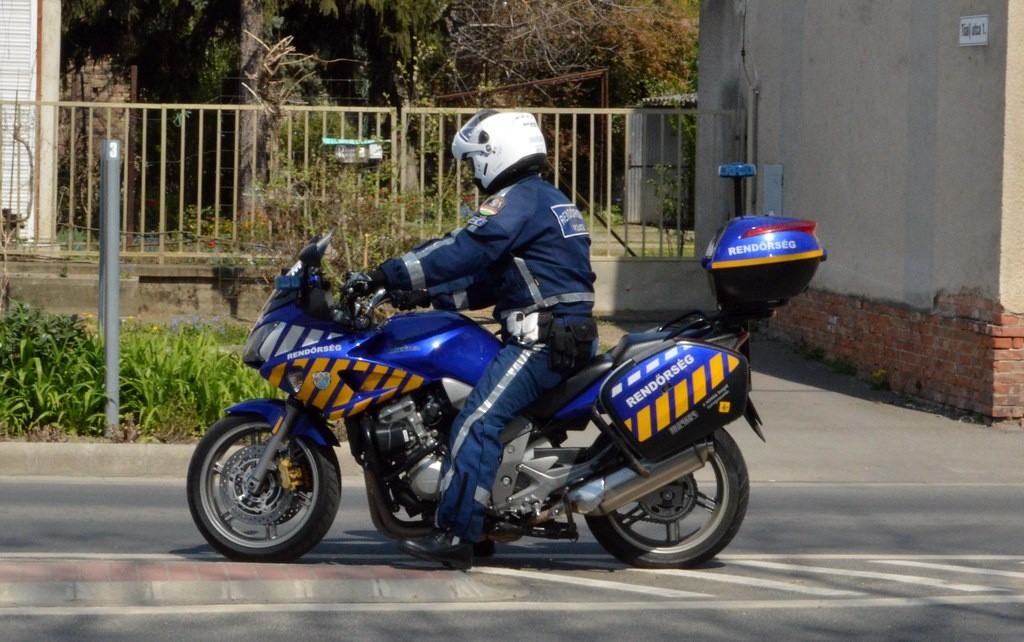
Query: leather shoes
[395,526,475,571]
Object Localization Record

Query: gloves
[340,266,390,303]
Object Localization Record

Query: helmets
[451,107,548,194]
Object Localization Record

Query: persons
[340,106,598,574]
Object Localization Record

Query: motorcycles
[187,163,827,568]
